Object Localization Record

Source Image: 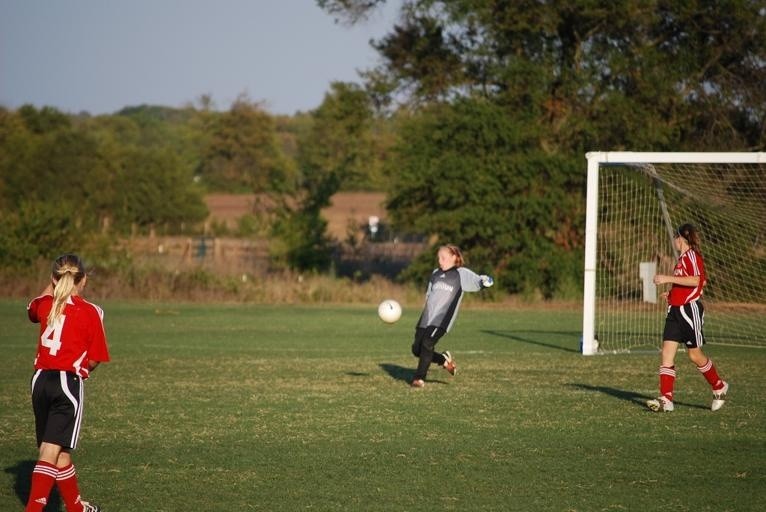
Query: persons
[410,242,495,387]
[24,255,110,512]
[644,223,730,413]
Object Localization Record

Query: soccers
[378,299,402,322]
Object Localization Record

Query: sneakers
[70,500,101,512]
[412,375,425,387]
[647,394,675,414]
[711,380,728,412]
[442,350,456,376]
